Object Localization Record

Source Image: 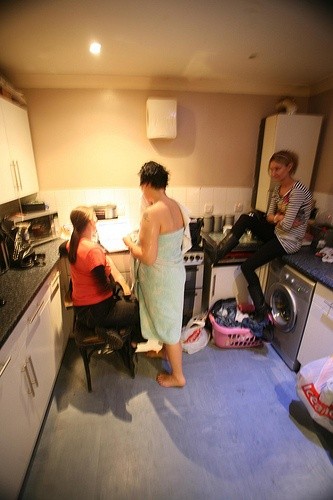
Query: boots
[204,232,239,264]
[247,280,272,320]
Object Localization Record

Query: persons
[127,159,188,389]
[65,205,149,363]
[201,147,314,323]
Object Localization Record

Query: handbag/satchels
[181,318,211,354]
[296,355,333,433]
[5,226,40,270]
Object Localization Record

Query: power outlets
[204,204,213,213]
[234,203,243,212]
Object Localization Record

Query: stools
[81,330,136,394]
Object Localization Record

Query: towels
[128,193,193,344]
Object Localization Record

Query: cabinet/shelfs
[295,282,333,370]
[0,258,71,500]
[65,253,130,340]
[0,98,40,205]
[203,252,270,314]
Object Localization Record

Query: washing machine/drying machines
[261,260,316,371]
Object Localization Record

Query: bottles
[313,227,328,255]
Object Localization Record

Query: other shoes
[96,327,123,350]
[123,344,137,357]
